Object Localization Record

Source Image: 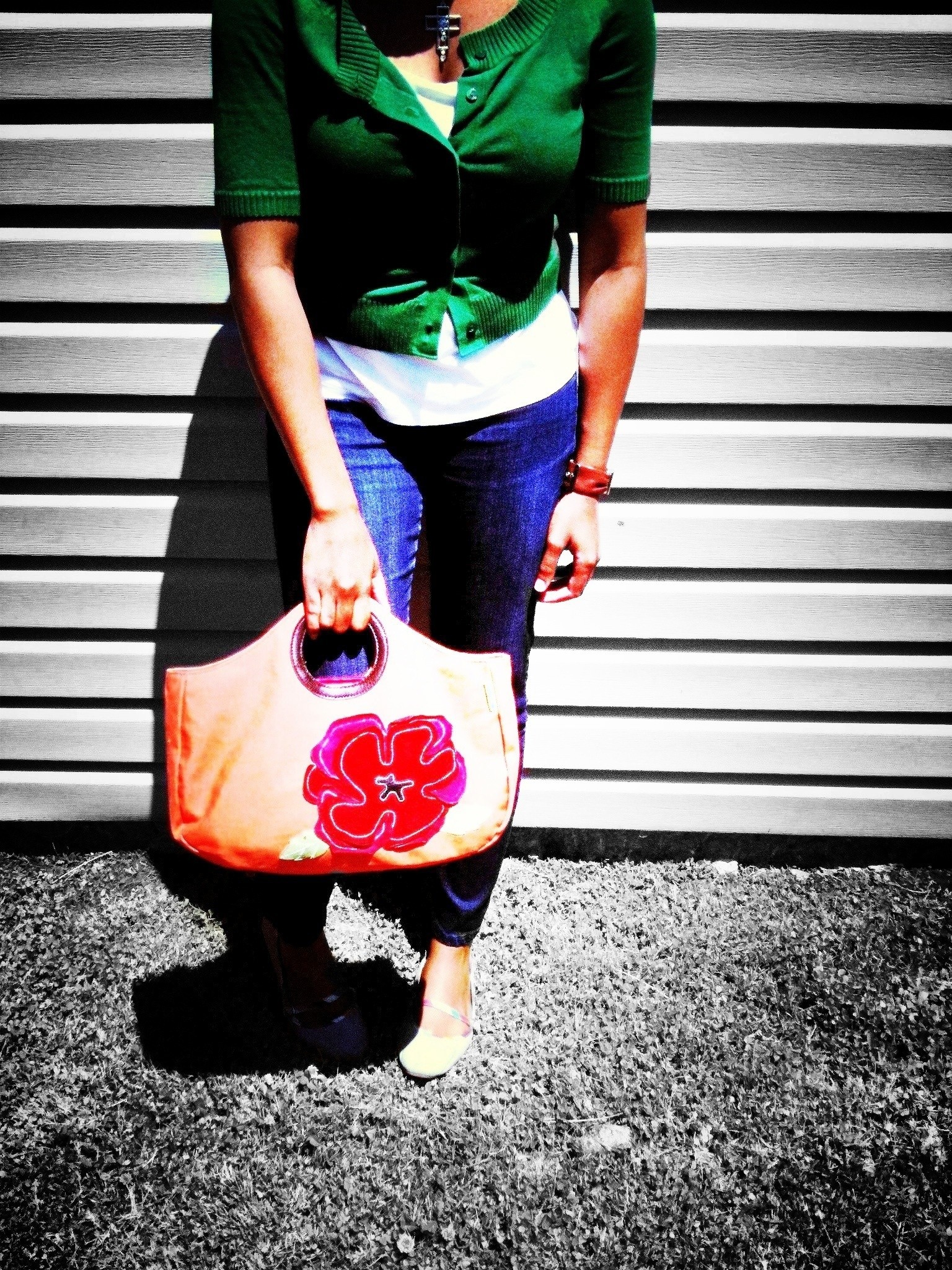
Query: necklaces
[425,1,461,62]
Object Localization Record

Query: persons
[211,0,656,1079]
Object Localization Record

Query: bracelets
[563,459,614,496]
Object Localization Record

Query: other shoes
[398,949,473,1078]
[262,917,367,1056]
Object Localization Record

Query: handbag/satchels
[164,598,520,876]
